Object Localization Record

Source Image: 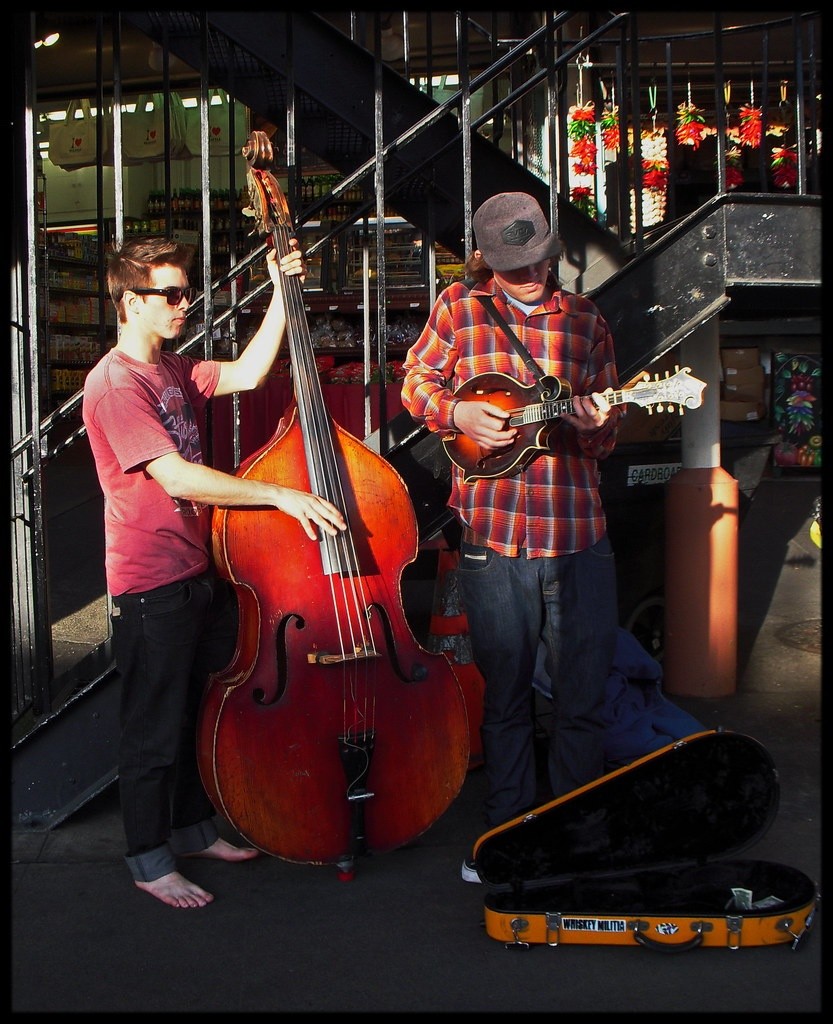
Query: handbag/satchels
[49,99,108,169]
[185,89,247,156]
[122,91,186,162]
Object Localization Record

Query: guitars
[440,364,708,486]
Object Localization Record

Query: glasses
[116,286,196,305]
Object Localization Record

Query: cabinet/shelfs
[34,197,463,425]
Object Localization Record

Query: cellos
[191,130,474,863]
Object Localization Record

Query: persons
[81,237,347,907]
[399,193,627,882]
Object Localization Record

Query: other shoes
[462,857,482,883]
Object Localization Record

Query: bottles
[181,215,250,230]
[295,175,364,200]
[147,185,249,212]
[309,204,352,221]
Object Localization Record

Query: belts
[461,525,527,548]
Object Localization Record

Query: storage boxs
[719,350,766,420]
[619,351,682,443]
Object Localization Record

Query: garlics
[629,136,668,235]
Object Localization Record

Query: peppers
[774,442,821,467]
[786,392,817,435]
[568,106,798,206]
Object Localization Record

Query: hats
[473,192,561,272]
